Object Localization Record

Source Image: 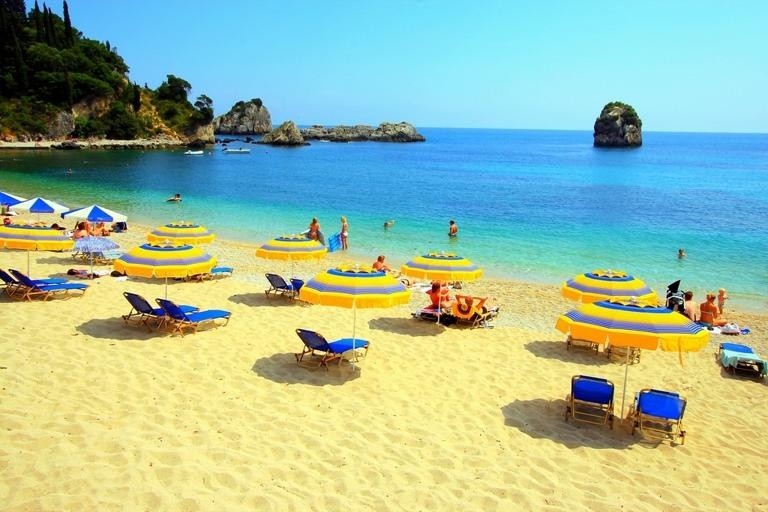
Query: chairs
[189,267,232,283]
[72,247,125,265]
[413,304,493,330]
[9,269,89,302]
[0,268,68,296]
[719,343,768,382]
[122,292,200,333]
[627,389,687,447]
[564,374,614,431]
[295,328,370,371]
[156,298,232,338]
[265,273,310,306]
[567,334,641,366]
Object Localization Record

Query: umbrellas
[143,220,215,249]
[75,236,120,272]
[113,240,218,327]
[400,250,482,328]
[0,222,75,278]
[255,232,326,299]
[0,191,28,205]
[299,261,412,370]
[554,300,709,424]
[61,204,128,231]
[9,197,70,223]
[561,269,663,309]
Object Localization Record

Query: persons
[373,255,391,272]
[426,282,455,308]
[166,193,182,202]
[448,221,458,236]
[677,248,686,257]
[309,216,320,241]
[455,294,499,313]
[67,269,127,279]
[72,221,127,239]
[384,220,395,231]
[666,288,728,325]
[453,281,462,289]
[341,216,348,249]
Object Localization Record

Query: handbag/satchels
[722,322,740,334]
